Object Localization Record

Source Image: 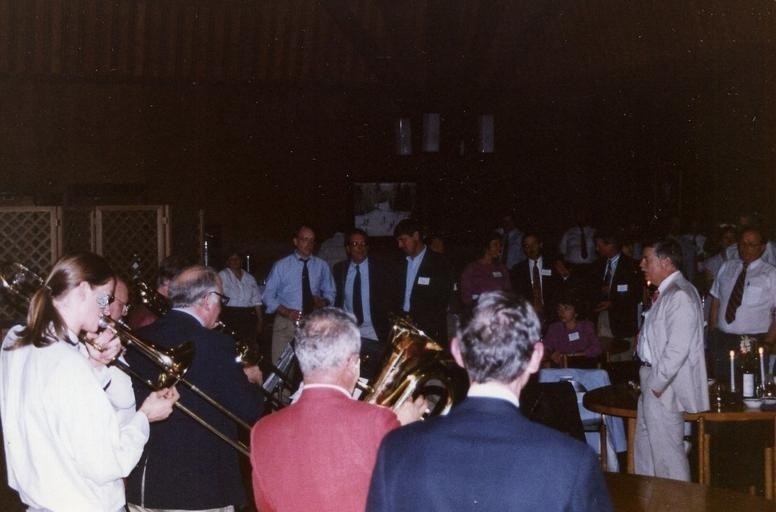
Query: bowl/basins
[762,396,776,406]
[743,398,764,408]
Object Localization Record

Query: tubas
[358,314,466,428]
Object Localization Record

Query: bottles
[742,356,755,398]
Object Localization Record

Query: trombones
[134,278,297,408]
[0,261,252,458]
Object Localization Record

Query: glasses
[209,291,230,305]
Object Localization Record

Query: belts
[639,361,652,367]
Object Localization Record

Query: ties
[533,261,542,312]
[300,258,314,315]
[353,265,365,327]
[724,263,748,324]
[580,225,589,259]
[604,260,612,287]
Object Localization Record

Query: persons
[83,203,775,475]
[0,253,179,512]
[119,267,262,511]
[364,288,614,511]
[250,305,402,512]
[631,239,711,482]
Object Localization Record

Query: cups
[766,374,774,395]
[712,383,727,407]
